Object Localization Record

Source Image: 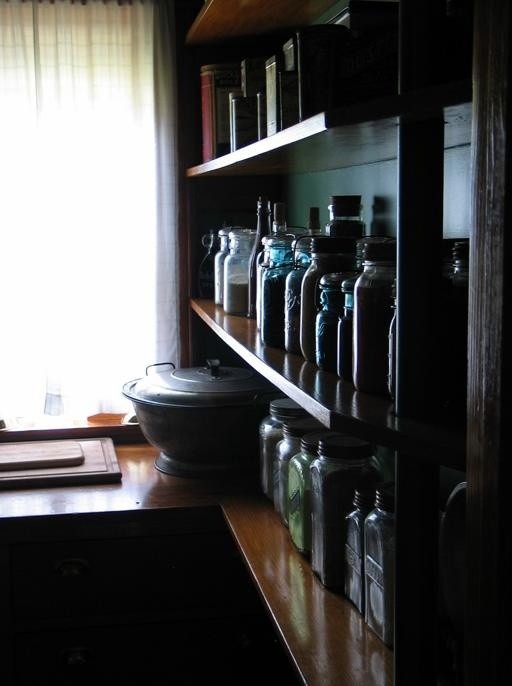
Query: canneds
[353,236,397,391]
[314,272,351,370]
[284,237,312,352]
[274,416,326,529]
[336,278,355,380]
[299,238,356,363]
[288,428,330,555]
[260,399,308,501]
[346,488,373,610]
[261,237,293,347]
[387,277,397,396]
[364,482,396,645]
[312,436,382,586]
[257,236,279,330]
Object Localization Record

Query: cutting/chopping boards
[0,439,123,492]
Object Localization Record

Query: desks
[2,442,217,518]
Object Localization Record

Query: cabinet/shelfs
[177,2,508,684]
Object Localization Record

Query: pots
[118,357,289,482]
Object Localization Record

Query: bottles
[256,389,399,655]
[192,188,469,411]
